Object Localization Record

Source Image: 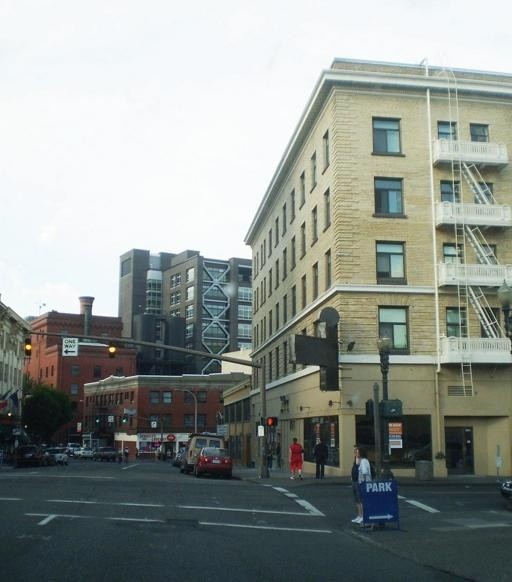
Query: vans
[182,435,225,475]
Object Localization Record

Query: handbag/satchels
[370,464,376,480]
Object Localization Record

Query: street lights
[174,387,198,434]
[376,338,396,479]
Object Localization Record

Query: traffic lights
[267,417,274,426]
[24,338,31,356]
[108,342,115,358]
[95,417,101,429]
[122,417,128,424]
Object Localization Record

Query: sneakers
[299,478,302,480]
[290,477,295,480]
[351,515,363,524]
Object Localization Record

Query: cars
[496,478,512,501]
[194,447,233,479]
[16,442,117,467]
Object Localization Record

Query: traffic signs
[361,480,399,523]
[62,338,78,357]
[150,422,157,429]
[107,416,114,423]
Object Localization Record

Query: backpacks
[320,446,328,459]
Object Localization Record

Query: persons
[135,447,140,460]
[118,448,123,464]
[355,448,374,483]
[276,442,282,468]
[166,448,172,462]
[288,437,306,481]
[266,441,274,472]
[312,437,329,479]
[124,446,129,464]
[351,444,364,524]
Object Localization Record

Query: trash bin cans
[415,460,433,481]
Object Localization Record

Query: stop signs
[167,435,175,441]
[154,442,160,448]
